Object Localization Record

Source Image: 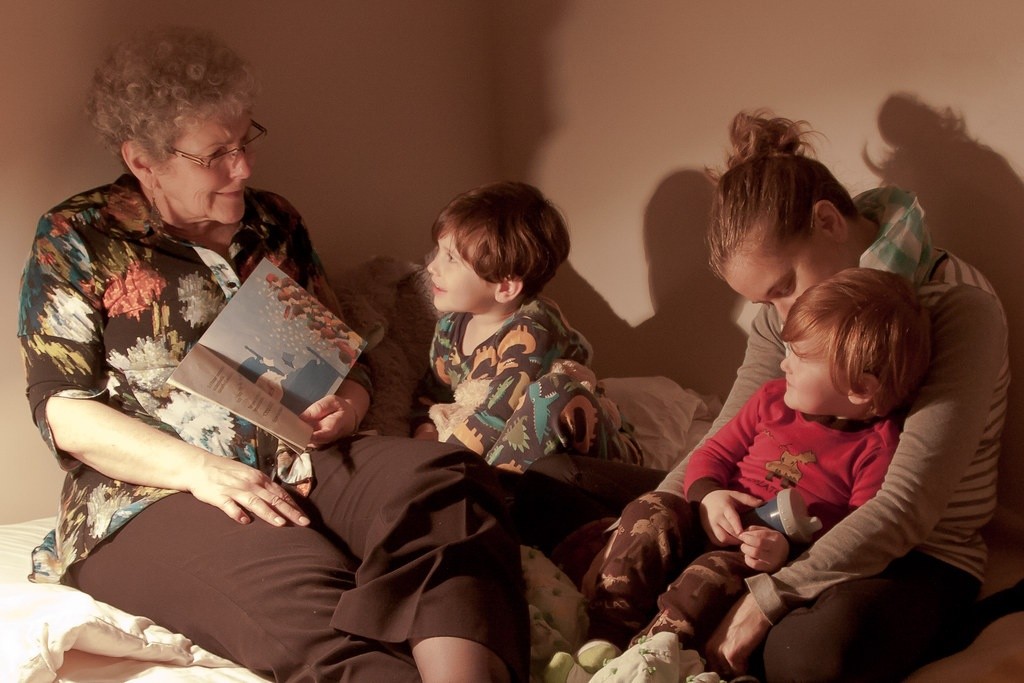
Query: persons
[576,268,938,676]
[408,177,646,575]
[515,110,1014,683]
[18,29,536,683]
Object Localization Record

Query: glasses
[167,119,267,173]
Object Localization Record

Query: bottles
[740,488,823,545]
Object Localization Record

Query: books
[164,256,368,460]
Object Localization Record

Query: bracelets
[353,411,361,432]
